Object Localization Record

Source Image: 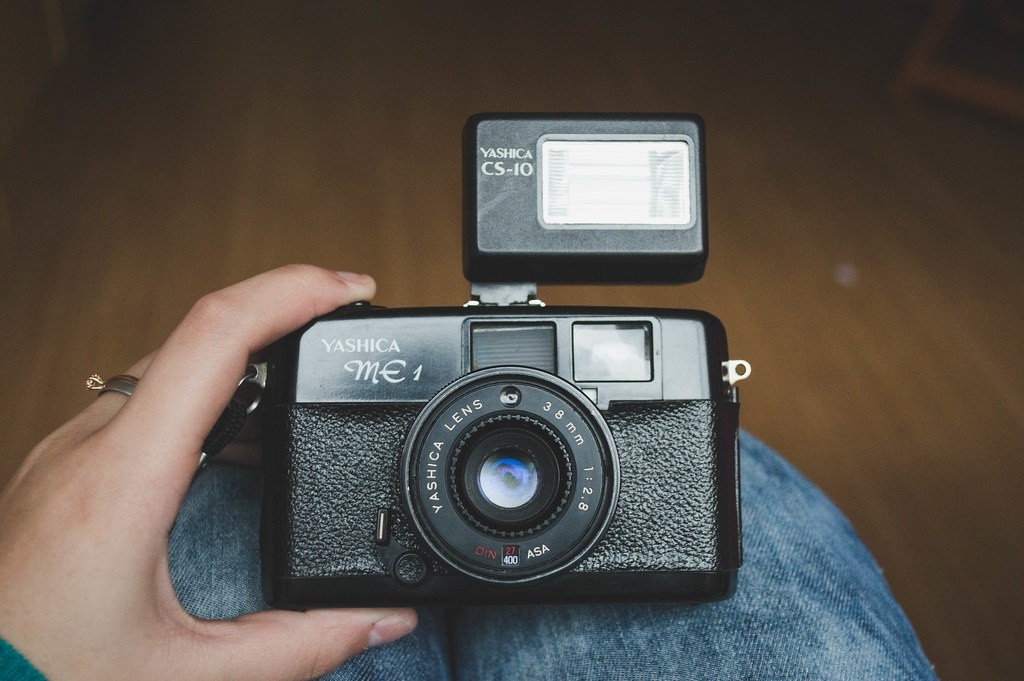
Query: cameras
[263,110,746,604]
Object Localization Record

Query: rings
[84,371,140,398]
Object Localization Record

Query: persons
[1,264,934,680]
